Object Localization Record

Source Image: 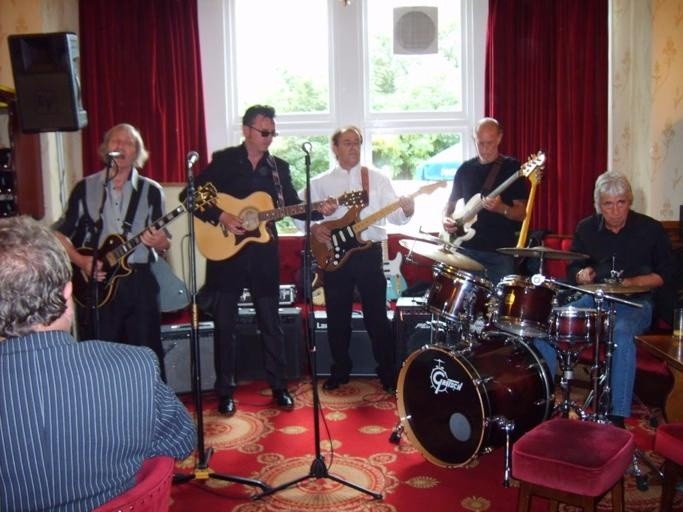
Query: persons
[177,105,340,419]
[1,214,198,511]
[441,117,528,318]
[533,170,675,430]
[53,122,171,385]
[290,123,415,395]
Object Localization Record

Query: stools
[653,421,682,511]
[510,417,637,511]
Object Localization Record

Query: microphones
[185,147,199,171]
[531,270,545,290]
[105,149,123,161]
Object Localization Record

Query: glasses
[250,126,276,137]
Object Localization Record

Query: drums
[492,274,558,339]
[396,330,557,469]
[553,307,616,343]
[421,262,494,325]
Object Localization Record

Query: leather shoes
[220,397,234,413]
[273,388,293,406]
[323,375,349,389]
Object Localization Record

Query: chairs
[85,450,176,510]
[525,218,680,397]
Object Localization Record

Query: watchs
[503,206,508,217]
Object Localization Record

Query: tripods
[251,280,384,508]
[586,373,642,489]
[172,299,270,498]
[551,370,587,423]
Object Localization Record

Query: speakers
[5,30,89,134]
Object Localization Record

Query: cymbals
[496,246,590,260]
[420,231,465,251]
[577,284,650,294]
[399,239,485,272]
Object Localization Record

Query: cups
[672,341,682,357]
[672,308,682,340]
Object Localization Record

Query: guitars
[439,150,545,251]
[194,191,369,262]
[514,151,545,258]
[71,182,218,309]
[312,266,327,307]
[381,240,408,301]
[312,180,447,272]
